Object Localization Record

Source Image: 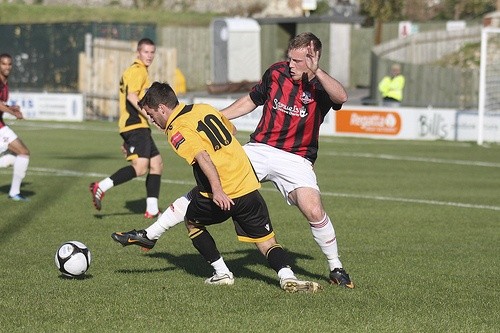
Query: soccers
[55,240,91,278]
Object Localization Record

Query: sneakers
[204,272,235,286]
[112,229,157,253]
[7,194,30,201]
[144,210,163,219]
[330,267,355,289]
[280,279,324,294]
[89,181,104,211]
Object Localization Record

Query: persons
[377,63,405,107]
[89,38,162,218]
[0,54,31,201]
[137,81,320,293]
[111,32,356,289]
